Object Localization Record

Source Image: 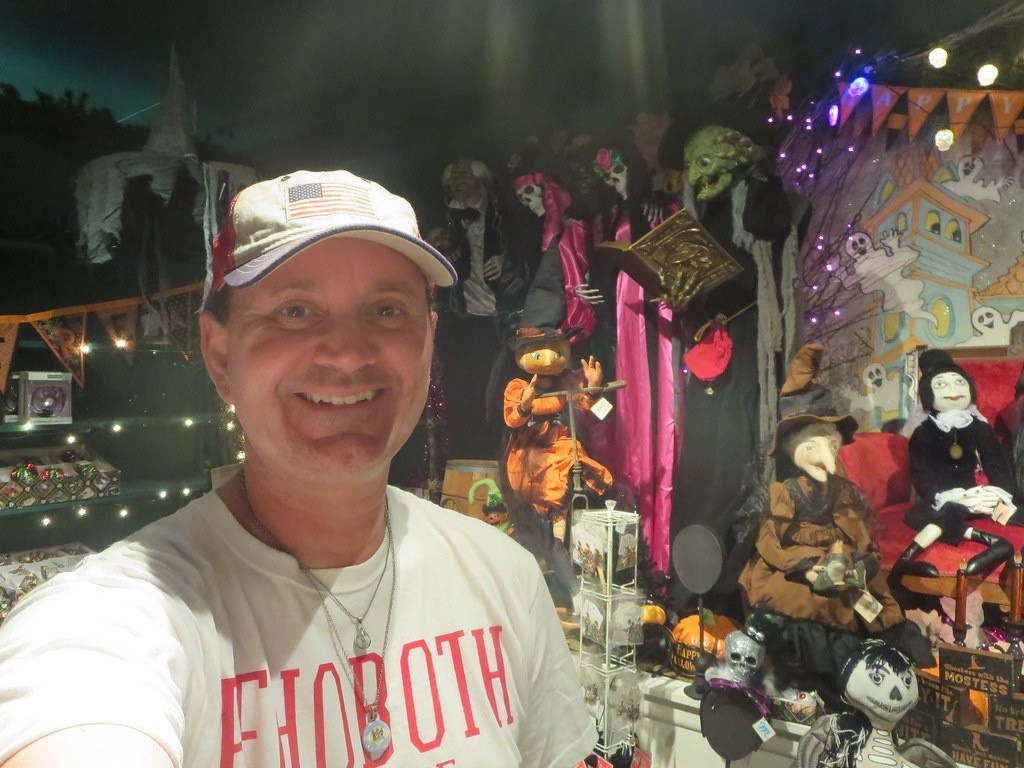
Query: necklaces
[237,461,392,649]
[234,459,399,762]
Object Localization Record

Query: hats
[209,169,459,292]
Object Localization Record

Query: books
[589,208,747,312]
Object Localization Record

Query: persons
[425,104,689,558]
[656,101,809,608]
[0,166,599,768]
[892,352,1019,575]
[484,304,615,583]
[736,408,938,711]
[798,639,960,768]
